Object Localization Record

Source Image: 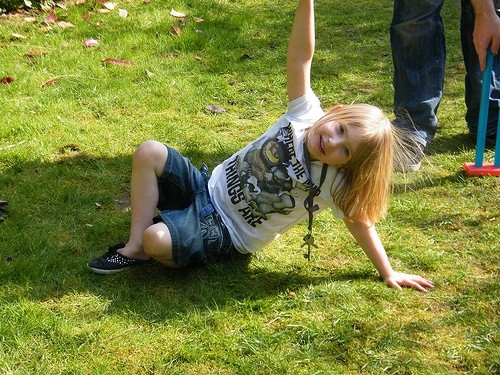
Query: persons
[391,0,500,171]
[87,0,435,293]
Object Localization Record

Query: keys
[300,234,318,261]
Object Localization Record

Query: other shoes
[393,138,425,170]
[86,243,151,274]
